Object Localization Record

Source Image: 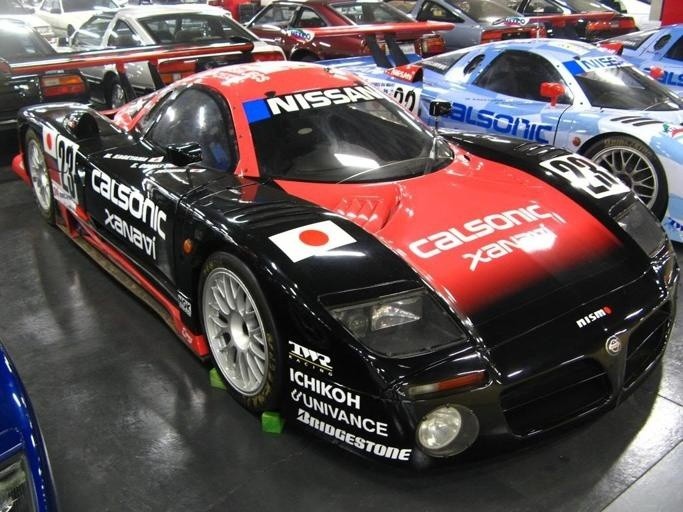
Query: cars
[489,0,637,43]
[239,0,455,63]
[0,0,273,52]
[587,22,683,98]
[0,17,91,168]
[57,2,287,112]
[311,36,683,244]
[384,0,548,53]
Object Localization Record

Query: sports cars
[0,36,681,474]
[250,18,682,244]
[0,340,59,512]
